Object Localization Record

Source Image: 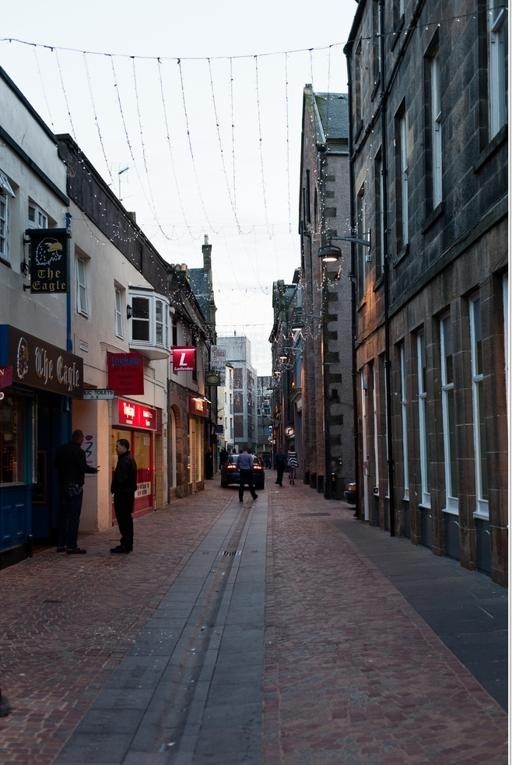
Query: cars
[221,454,264,489]
[344,479,356,504]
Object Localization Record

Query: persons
[57,428,100,556]
[274,446,287,488]
[109,438,138,555]
[235,446,259,503]
[287,443,301,488]
[219,445,272,471]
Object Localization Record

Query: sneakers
[58,544,133,555]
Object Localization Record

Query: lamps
[318,228,371,263]
[127,304,132,320]
